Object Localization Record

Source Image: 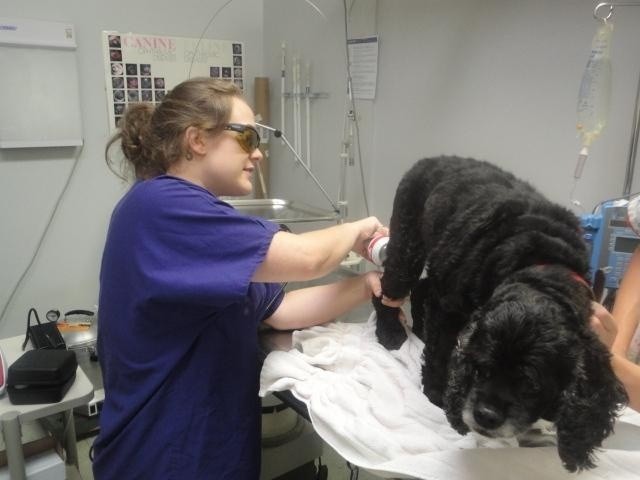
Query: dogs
[370,154,631,475]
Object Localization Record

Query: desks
[0,335,93,479]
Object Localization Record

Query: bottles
[362,236,391,266]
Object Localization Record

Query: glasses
[223,124,260,153]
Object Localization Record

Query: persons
[89,75,405,480]
[585,193,640,412]
